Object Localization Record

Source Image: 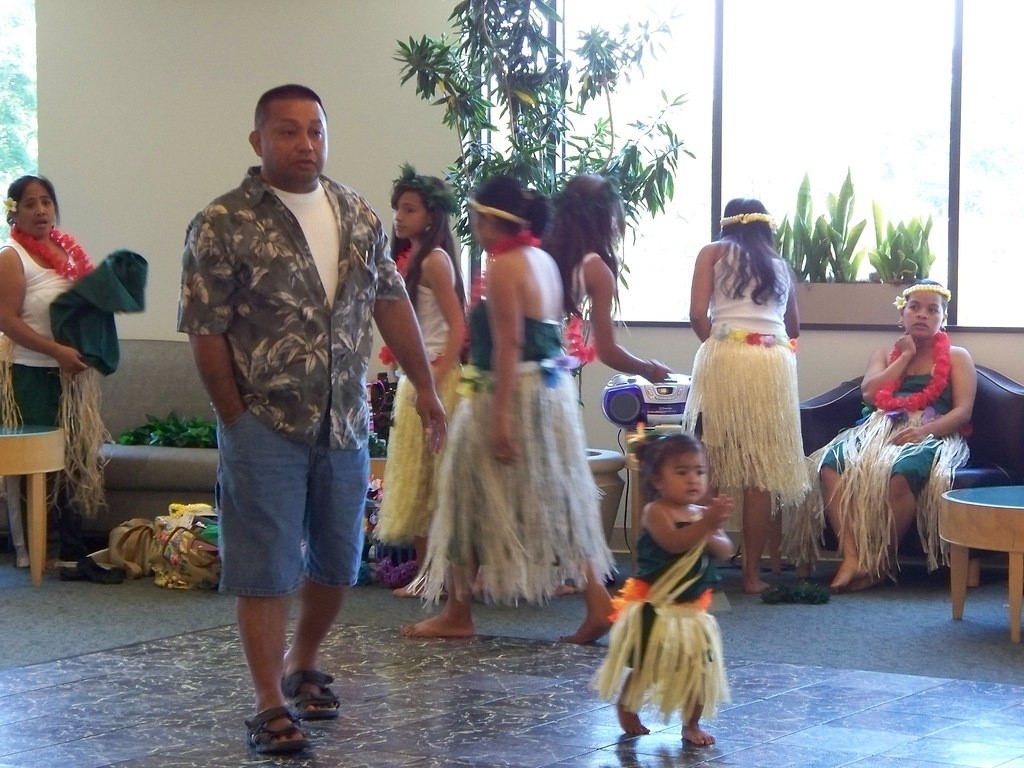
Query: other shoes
[58,542,98,562]
[60,557,123,584]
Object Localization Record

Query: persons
[588,435,734,746]
[371,173,467,597]
[401,174,620,644]
[809,282,978,591]
[681,199,812,593]
[176,84,448,755]
[0,175,90,569]
[539,174,672,595]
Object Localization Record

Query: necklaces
[461,230,542,350]
[567,307,596,363]
[378,248,414,364]
[9,226,94,283]
[873,330,951,412]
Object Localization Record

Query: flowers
[716,321,799,354]
[892,295,907,310]
[378,344,397,365]
[567,313,598,364]
[456,366,489,400]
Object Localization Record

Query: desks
[940,486,1024,644]
[0,423,67,588]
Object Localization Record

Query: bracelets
[649,361,658,384]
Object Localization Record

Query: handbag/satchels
[190,516,219,548]
[108,519,155,578]
[154,526,222,589]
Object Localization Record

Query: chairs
[800,364,1024,589]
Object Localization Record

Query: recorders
[600,372,693,428]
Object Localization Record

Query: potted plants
[772,166,937,326]
[391,0,696,544]
[82,409,388,550]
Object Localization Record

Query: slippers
[244,705,310,754]
[281,670,340,718]
